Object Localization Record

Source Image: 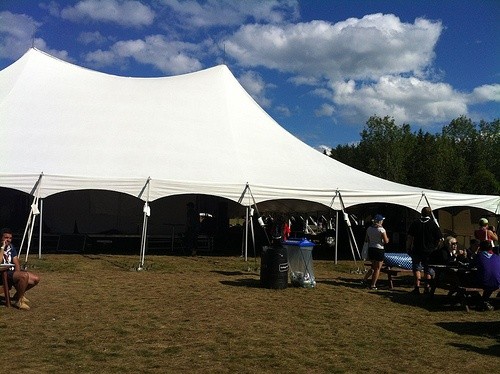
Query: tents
[0,48,500,271]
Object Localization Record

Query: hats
[479,218,489,223]
[374,214,385,221]
[470,239,480,245]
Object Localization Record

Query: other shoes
[370,285,379,290]
[360,280,369,287]
[409,287,430,296]
[13,298,30,311]
[12,293,29,302]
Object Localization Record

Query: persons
[361,214,389,290]
[271,214,289,247]
[0,229,40,309]
[430,234,500,311]
[407,206,444,296]
[186,202,201,255]
[314,223,329,260]
[474,218,498,248]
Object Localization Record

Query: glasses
[452,242,457,245]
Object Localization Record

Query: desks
[427,264,479,313]
[0,266,11,309]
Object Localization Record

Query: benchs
[419,278,434,287]
[457,287,500,297]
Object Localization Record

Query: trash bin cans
[260,247,288,288]
[280,236,317,289]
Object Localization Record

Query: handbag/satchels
[485,229,495,248]
[361,234,372,261]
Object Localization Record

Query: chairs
[380,253,413,290]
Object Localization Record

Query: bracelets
[1,246,4,249]
[493,231,496,233]
